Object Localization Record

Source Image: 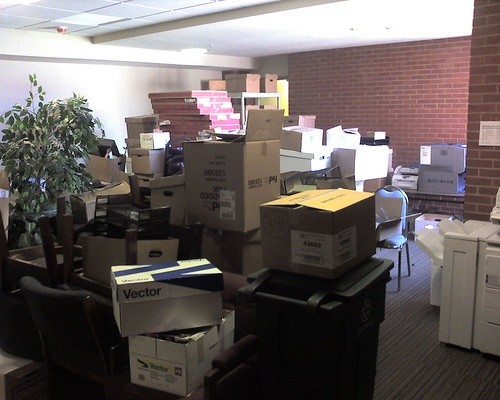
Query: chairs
[371,185,411,292]
[19,276,129,400]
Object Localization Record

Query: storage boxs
[0,72,391,398]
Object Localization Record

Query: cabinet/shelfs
[439,220,500,358]
